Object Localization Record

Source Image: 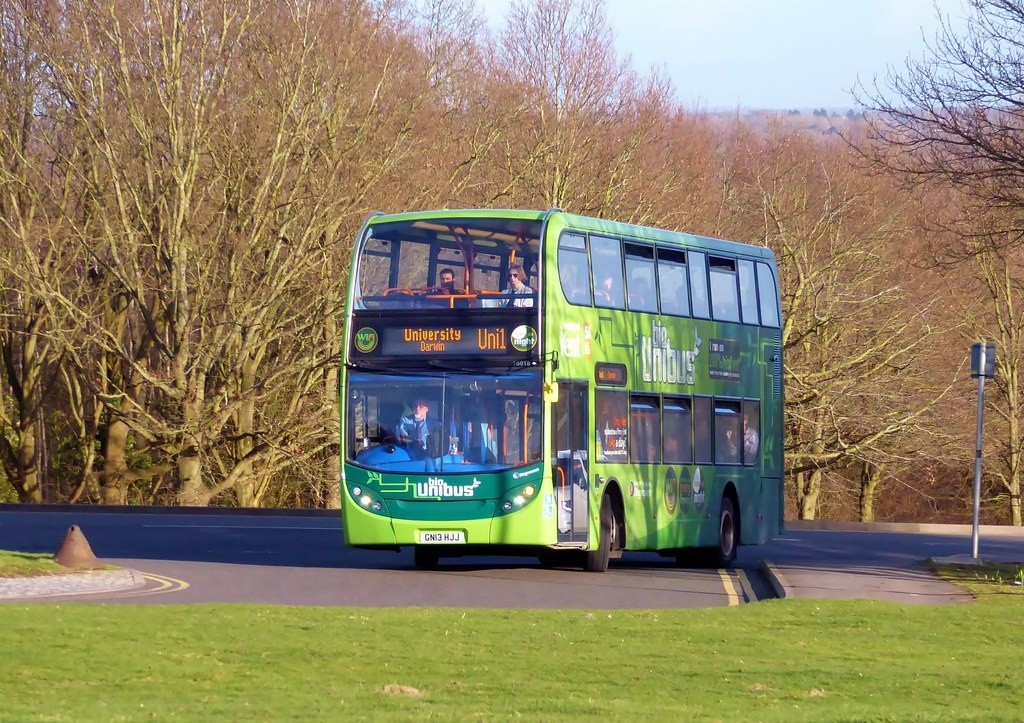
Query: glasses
[508,274,519,278]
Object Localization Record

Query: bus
[337,207,785,573]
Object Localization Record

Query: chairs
[596,289,611,302]
[383,288,468,297]
[629,293,645,306]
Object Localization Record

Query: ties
[415,420,425,447]
[506,290,517,308]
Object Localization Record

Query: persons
[388,261,769,461]
